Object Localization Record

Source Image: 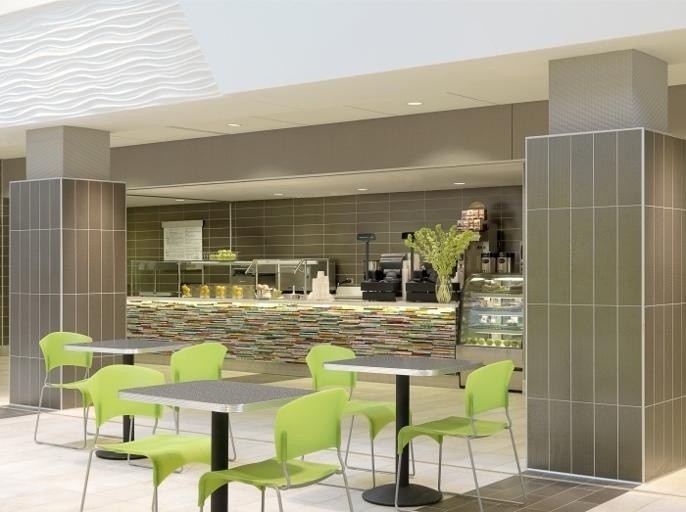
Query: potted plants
[399,222,483,299]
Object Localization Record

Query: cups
[181,283,244,300]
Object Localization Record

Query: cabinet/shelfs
[456,272,529,394]
